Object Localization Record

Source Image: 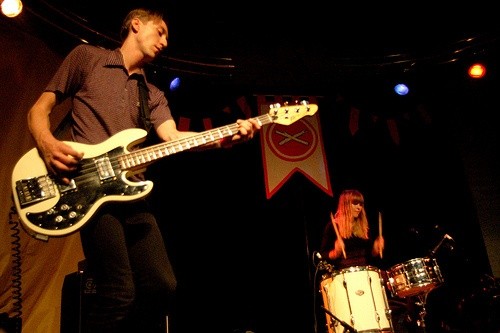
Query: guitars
[11,100,318,237]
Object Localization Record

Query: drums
[387,258,444,298]
[319,266,395,333]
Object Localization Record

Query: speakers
[60,271,98,333]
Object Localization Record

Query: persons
[27,8,261,333]
[322,189,385,270]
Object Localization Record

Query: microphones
[316,252,336,277]
[431,235,446,256]
